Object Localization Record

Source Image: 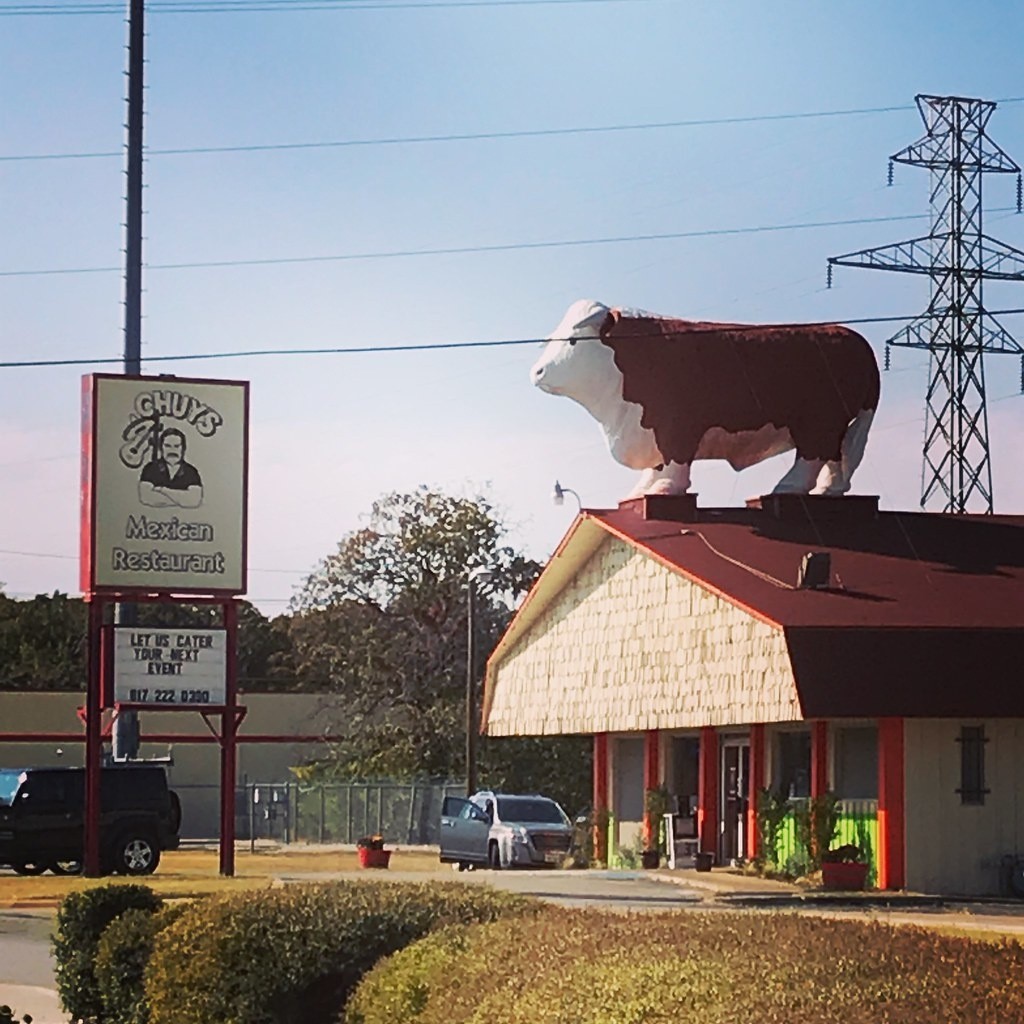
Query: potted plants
[638,783,671,869]
[821,844,869,892]
[356,834,393,868]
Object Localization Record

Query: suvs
[0,765,181,878]
[437,787,578,873]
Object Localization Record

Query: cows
[531,298,882,494]
